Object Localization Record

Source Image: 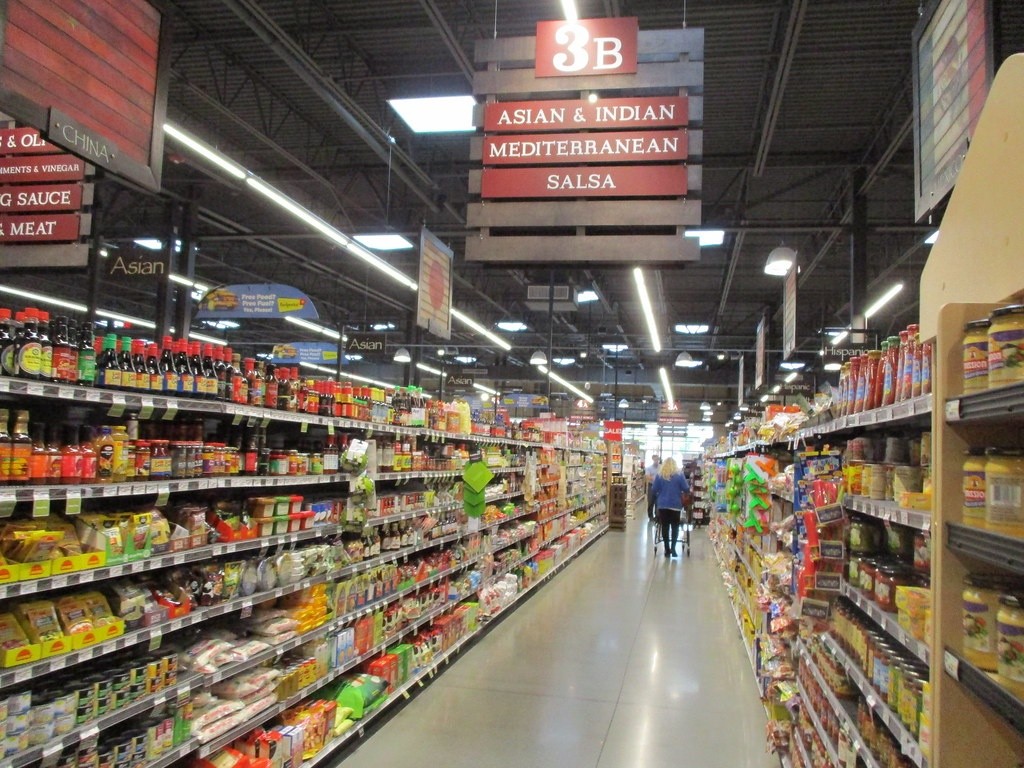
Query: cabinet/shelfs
[696,300,1024,768]
[0,304,645,768]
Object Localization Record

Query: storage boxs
[253,518,275,537]
[49,547,81,575]
[274,515,290,534]
[169,531,208,551]
[533,550,554,575]
[454,601,480,633]
[249,497,276,517]
[289,514,303,532]
[0,558,17,583]
[356,658,378,673]
[300,511,316,530]
[31,636,72,658]
[898,610,925,638]
[289,494,303,514]
[0,641,41,668]
[68,616,101,649]
[443,614,463,643]
[381,654,398,691]
[96,617,125,640]
[433,614,449,650]
[895,585,930,617]
[389,644,412,685]
[65,544,105,569]
[547,540,570,565]
[7,557,52,580]
[370,661,390,696]
[274,497,291,516]
[565,532,580,552]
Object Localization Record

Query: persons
[684,463,690,480]
[648,457,690,557]
[646,455,662,522]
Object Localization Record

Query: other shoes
[672,553,677,556]
[666,550,671,556]
[650,518,654,521]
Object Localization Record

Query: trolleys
[651,498,691,559]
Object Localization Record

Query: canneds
[138,415,323,479]
[0,650,192,768]
[788,304,1024,768]
[421,441,454,471]
[306,490,426,523]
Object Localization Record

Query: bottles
[0,307,558,562]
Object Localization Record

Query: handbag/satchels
[681,492,693,507]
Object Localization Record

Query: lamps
[700,401,711,411]
[392,346,411,364]
[702,416,712,421]
[831,283,906,345]
[351,232,416,253]
[704,410,714,416]
[162,118,512,353]
[618,399,629,409]
[578,279,599,303]
[601,336,629,352]
[415,362,500,397]
[529,347,547,365]
[344,352,363,361]
[675,349,703,368]
[539,365,594,405]
[497,300,527,331]
[764,246,794,278]
